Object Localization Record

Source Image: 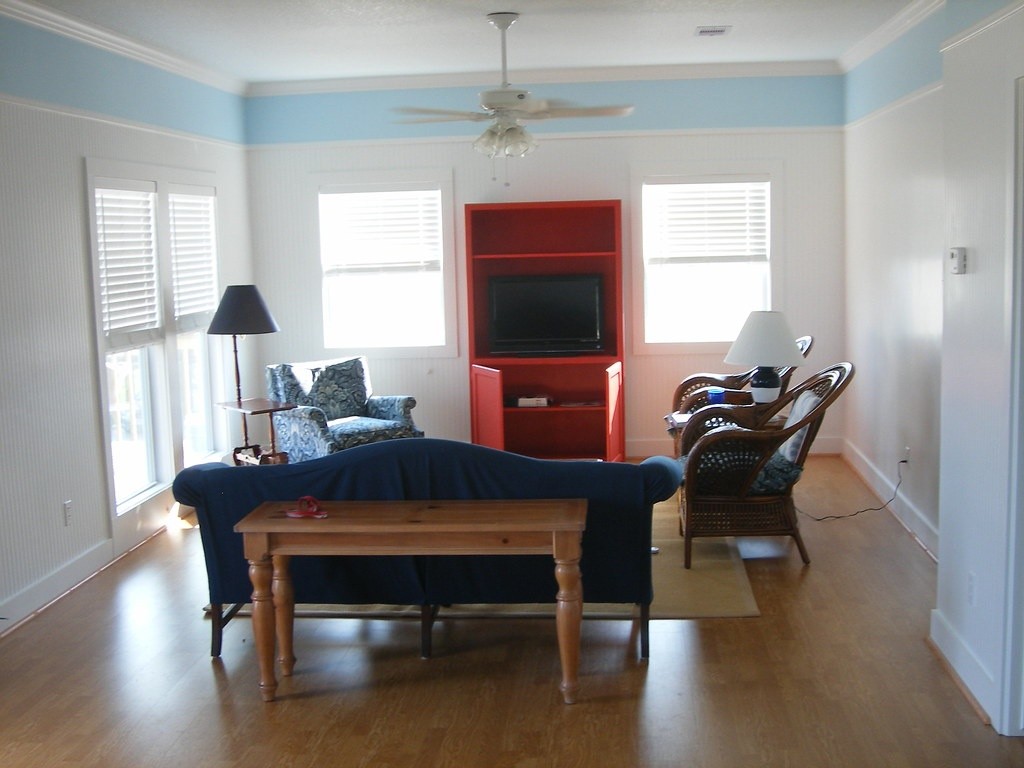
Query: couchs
[265,354,427,467]
[171,438,685,662]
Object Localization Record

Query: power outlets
[904,446,911,467]
[64,499,74,526]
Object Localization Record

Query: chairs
[672,334,813,461]
[678,360,855,571]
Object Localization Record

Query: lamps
[723,310,806,415]
[471,116,541,188]
[207,283,281,470]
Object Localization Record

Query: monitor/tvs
[487,273,606,357]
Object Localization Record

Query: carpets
[202,487,763,618]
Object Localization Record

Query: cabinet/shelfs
[463,199,625,358]
[468,359,627,462]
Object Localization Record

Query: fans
[387,10,638,126]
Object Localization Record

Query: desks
[674,413,788,455]
[233,498,589,703]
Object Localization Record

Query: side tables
[219,396,300,467]
